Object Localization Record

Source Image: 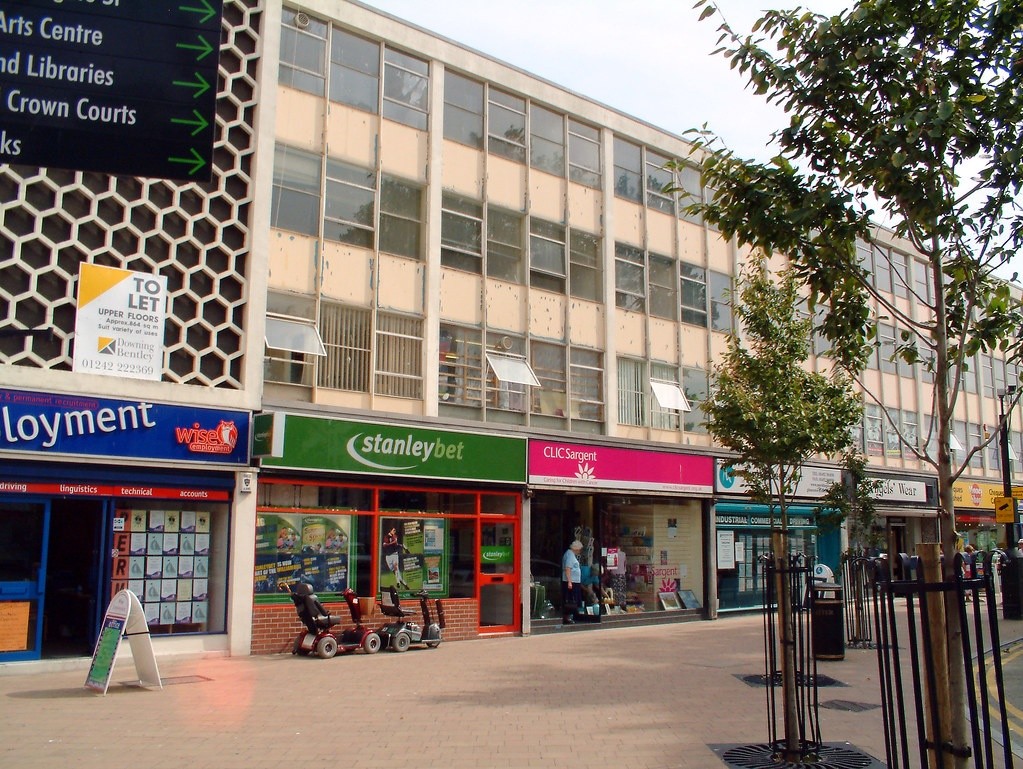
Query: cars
[530,555,564,607]
[342,540,371,565]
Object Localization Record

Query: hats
[1018,539,1023,544]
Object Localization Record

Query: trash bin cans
[814,584,846,663]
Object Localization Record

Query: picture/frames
[677,590,702,609]
[658,592,682,611]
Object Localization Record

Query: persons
[962,544,977,601]
[383,525,410,590]
[277,526,346,552]
[608,546,626,611]
[1016,539,1023,558]
[562,540,583,624]
[581,564,608,604]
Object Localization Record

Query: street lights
[995,383,1019,553]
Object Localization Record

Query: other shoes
[563,619,575,625]
[405,585,409,589]
[396,583,400,588]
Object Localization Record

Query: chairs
[379,585,417,617]
[295,583,341,625]
